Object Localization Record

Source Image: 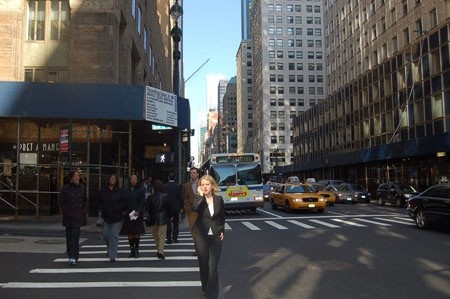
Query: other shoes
[172,239,179,243]
[111,257,115,263]
[165,240,171,244]
[70,257,76,264]
[157,253,164,259]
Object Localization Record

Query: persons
[59,171,87,264]
[184,167,202,254]
[191,174,225,299]
[121,174,145,258]
[98,173,125,263]
[161,173,183,244]
[146,180,171,261]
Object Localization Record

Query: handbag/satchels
[146,212,155,227]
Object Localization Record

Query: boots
[129,247,135,258]
[135,246,138,258]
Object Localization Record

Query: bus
[198,152,264,213]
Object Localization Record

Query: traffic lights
[155,153,173,163]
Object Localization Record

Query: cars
[263,180,281,200]
[318,179,371,205]
[304,178,336,207]
[269,176,326,214]
[406,183,450,231]
[376,181,418,208]
